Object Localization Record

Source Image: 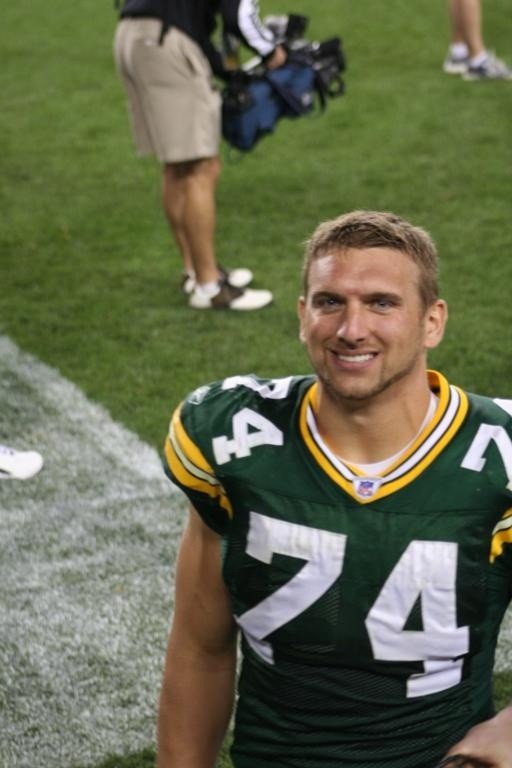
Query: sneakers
[443,44,512,82]
[182,266,272,311]
[0,446,42,479]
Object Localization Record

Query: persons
[442,1,512,81]
[112,1,288,312]
[155,207,511,768]
